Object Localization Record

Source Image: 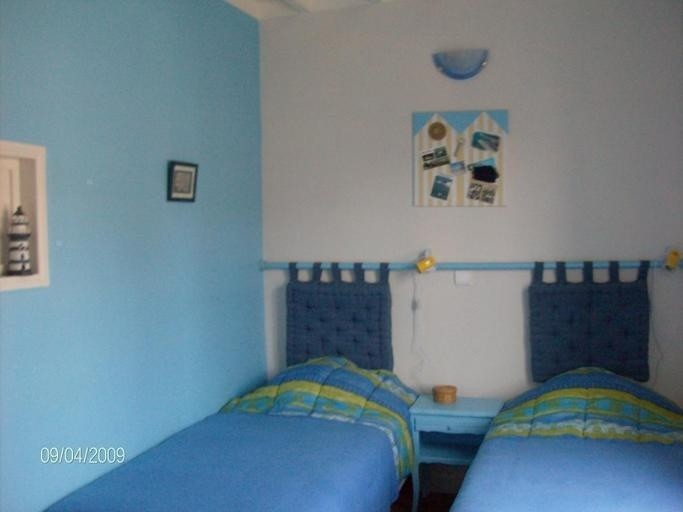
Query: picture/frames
[167,160,198,201]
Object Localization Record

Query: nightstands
[407,394,505,511]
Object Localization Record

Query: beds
[448,364,680,512]
[41,357,417,510]
